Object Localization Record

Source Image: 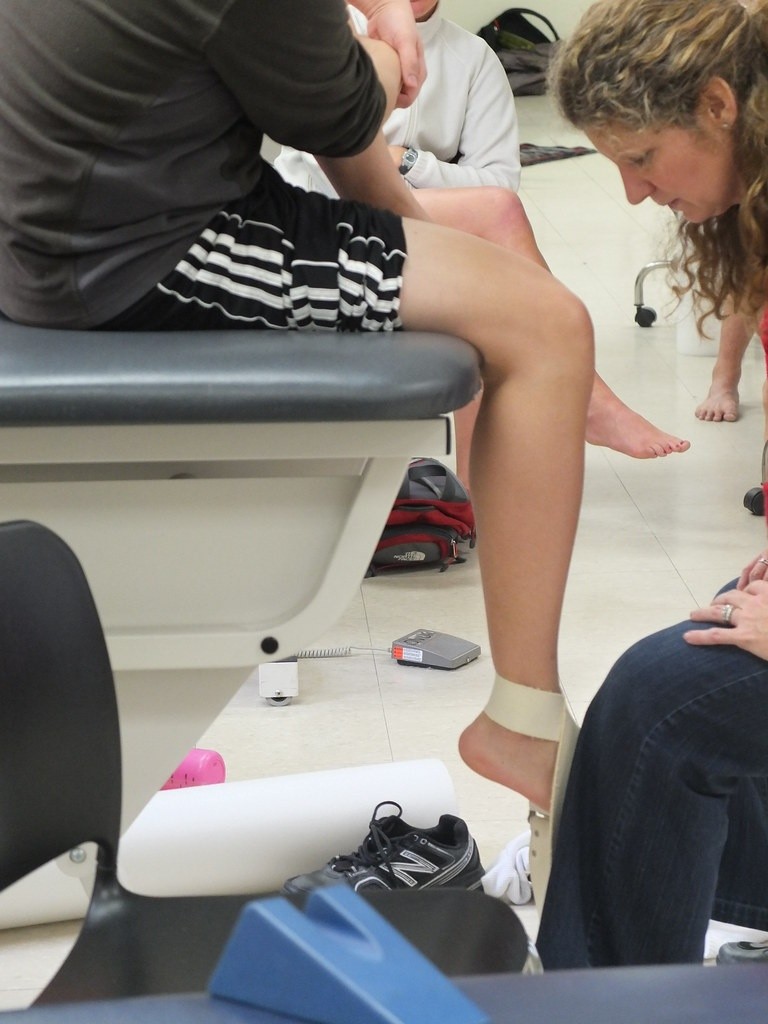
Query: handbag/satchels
[475,7,562,97]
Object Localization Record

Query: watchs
[398,148,418,175]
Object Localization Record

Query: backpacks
[369,459,475,576]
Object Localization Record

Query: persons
[0,0,691,810]
[280,0,767,977]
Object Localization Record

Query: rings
[759,558,768,565]
[720,605,733,624]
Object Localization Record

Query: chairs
[0,518,529,1012]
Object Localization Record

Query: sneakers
[718,937,768,964]
[285,798,484,904]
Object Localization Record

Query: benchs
[0,317,482,932]
